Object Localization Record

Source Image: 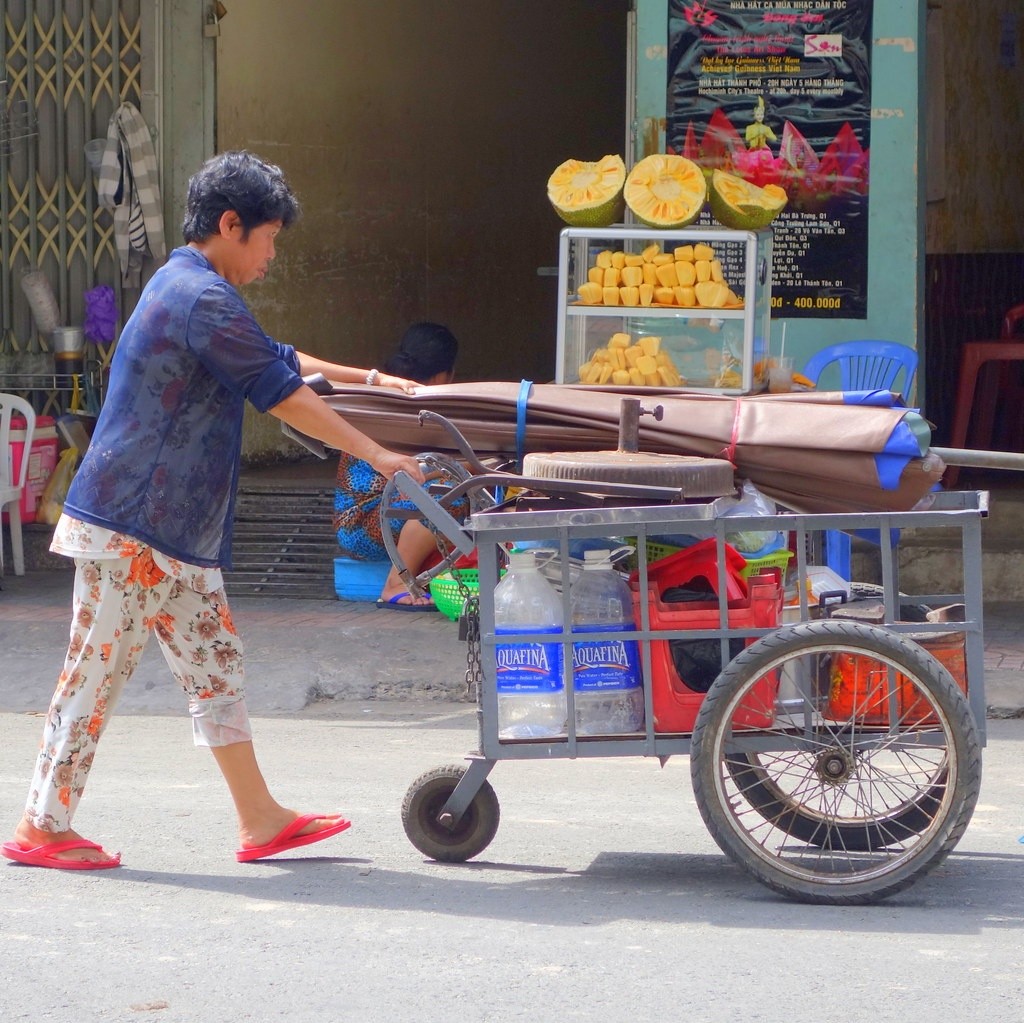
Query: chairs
[794,338,918,581]
[1,392,36,577]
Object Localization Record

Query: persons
[332,323,514,612]
[746,106,777,149]
[0,151,353,871]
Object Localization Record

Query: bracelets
[365,368,379,385]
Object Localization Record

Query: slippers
[3,831,120,870]
[377,591,438,612]
[235,808,352,864]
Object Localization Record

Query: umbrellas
[281,368,1024,549]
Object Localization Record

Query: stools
[624,536,783,730]
[941,339,1024,489]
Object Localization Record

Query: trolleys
[392,455,988,906]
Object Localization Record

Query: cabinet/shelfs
[554,223,774,394]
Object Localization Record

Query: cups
[768,357,795,393]
[83,137,107,177]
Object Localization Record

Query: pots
[777,591,849,715]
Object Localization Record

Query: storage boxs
[822,629,966,724]
[463,489,990,759]
[629,528,796,592]
[60,415,97,468]
[6,415,59,524]
[773,590,848,714]
[335,554,391,604]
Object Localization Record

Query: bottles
[571,546,645,733]
[493,548,563,738]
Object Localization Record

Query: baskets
[430,568,508,623]
[627,537,793,590]
